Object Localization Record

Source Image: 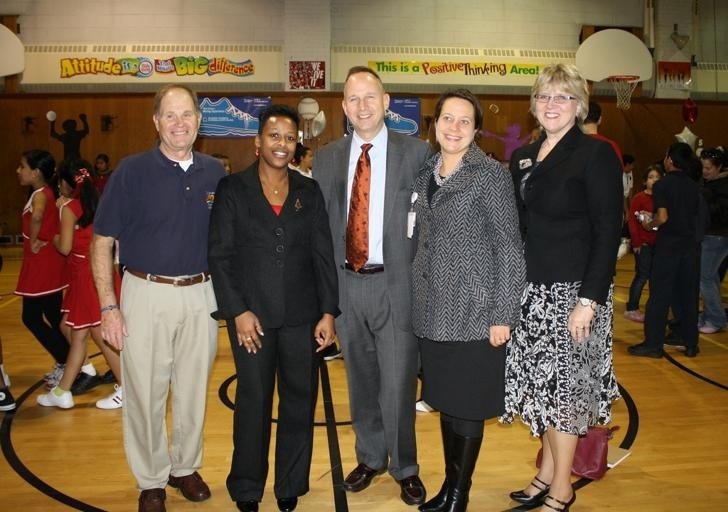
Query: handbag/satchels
[536,425,619,481]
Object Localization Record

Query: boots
[419,418,483,512]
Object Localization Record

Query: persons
[312,66,438,506]
[506,62,625,512]
[404,89,527,512]
[0,135,341,413]
[88,85,227,512]
[617,136,728,359]
[210,102,342,512]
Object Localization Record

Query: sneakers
[623,309,721,358]
[36,362,122,409]
[0,364,16,412]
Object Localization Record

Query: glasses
[533,94,576,103]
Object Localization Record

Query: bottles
[634,211,659,231]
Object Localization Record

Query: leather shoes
[138,488,166,512]
[509,477,550,505]
[236,502,258,512]
[277,497,297,512]
[395,474,426,505]
[168,471,210,501]
[344,463,377,492]
[544,488,576,512]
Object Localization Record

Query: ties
[346,143,373,272]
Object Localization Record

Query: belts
[126,267,210,286]
[345,264,384,274]
